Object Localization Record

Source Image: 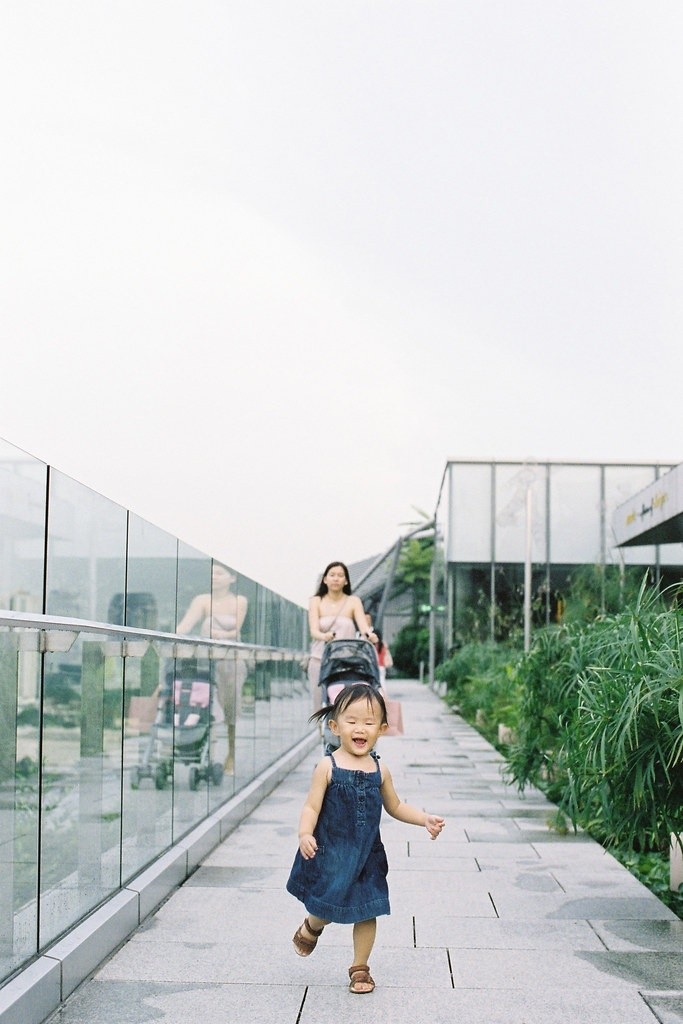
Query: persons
[308,561,378,726]
[173,559,247,776]
[372,630,388,701]
[355,608,377,640]
[285,683,443,996]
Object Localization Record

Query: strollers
[319,632,382,764]
[131,637,222,792]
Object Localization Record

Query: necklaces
[211,591,230,607]
[324,593,344,608]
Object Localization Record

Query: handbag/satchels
[378,687,402,736]
[385,651,394,668]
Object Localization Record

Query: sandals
[348,964,377,994]
[292,919,326,955]
